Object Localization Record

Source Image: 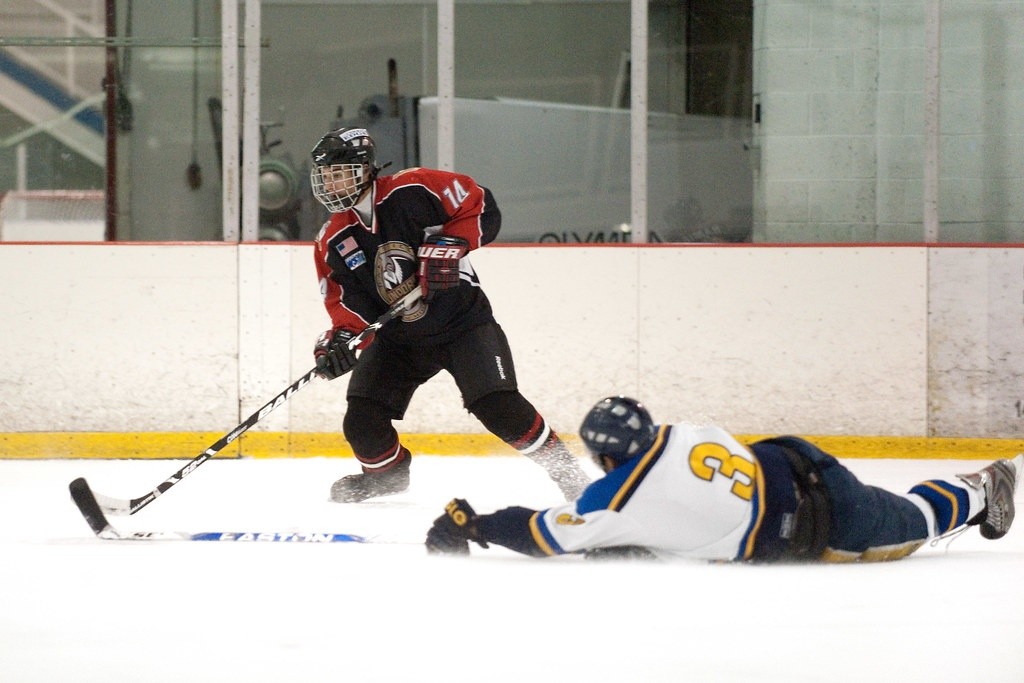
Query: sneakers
[955,454,1023,540]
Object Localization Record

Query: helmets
[579,395,657,457]
[311,126,377,209]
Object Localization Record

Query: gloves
[315,330,362,380]
[419,245,464,301]
[425,498,488,554]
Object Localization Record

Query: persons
[311,128,590,504]
[426,396,1016,564]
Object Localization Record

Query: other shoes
[560,472,590,500]
[331,468,410,503]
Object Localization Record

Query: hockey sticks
[71,286,422,516]
[68,478,369,543]
